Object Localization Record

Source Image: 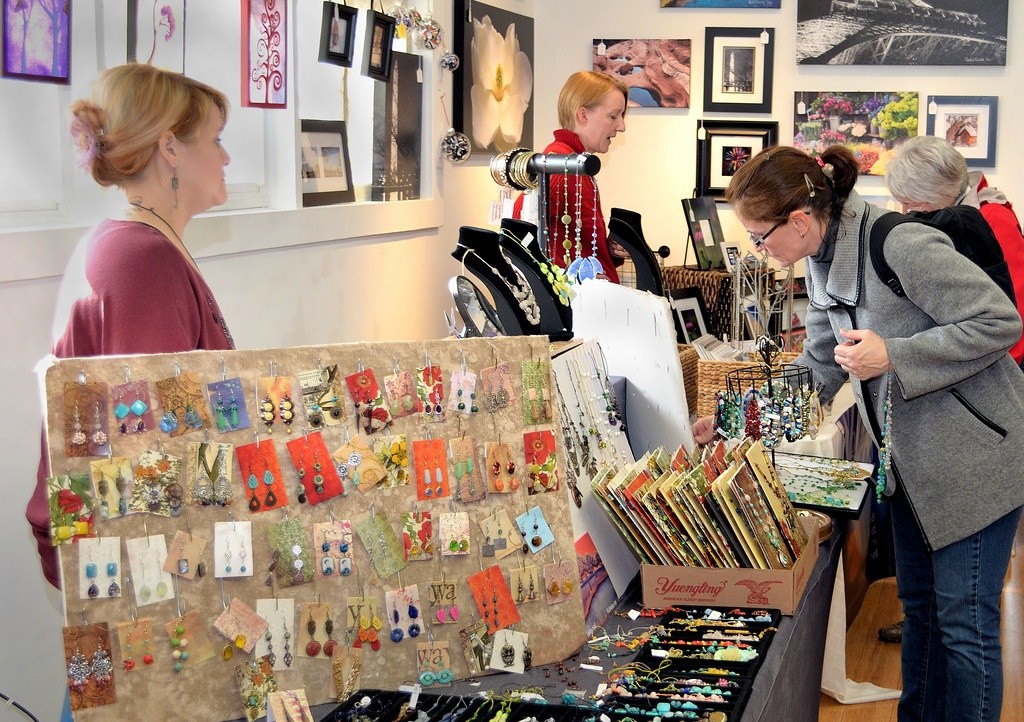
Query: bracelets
[409,682,598,721]
[713,386,822,447]
[490,148,538,191]
[586,603,777,722]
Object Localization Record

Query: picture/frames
[694,119,780,203]
[681,197,725,270]
[318,2,360,71]
[926,95,998,167]
[300,118,355,208]
[127,1,187,78]
[719,240,743,273]
[667,285,713,345]
[1,1,73,86]
[361,8,396,83]
[702,26,776,112]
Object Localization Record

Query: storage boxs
[639,515,820,615]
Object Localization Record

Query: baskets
[696,351,800,417]
[677,342,699,420]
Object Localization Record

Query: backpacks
[869,204,1017,307]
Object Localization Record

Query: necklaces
[129,201,201,271]
[552,343,628,508]
[456,242,540,325]
[767,454,870,509]
[594,444,794,567]
[876,359,892,504]
[541,152,597,304]
[500,227,569,306]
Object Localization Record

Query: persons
[878,136,1024,639]
[28,64,236,722]
[691,145,1024,722]
[513,71,630,285]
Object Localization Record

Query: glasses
[750,212,812,251]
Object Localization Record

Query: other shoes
[879,621,903,643]
